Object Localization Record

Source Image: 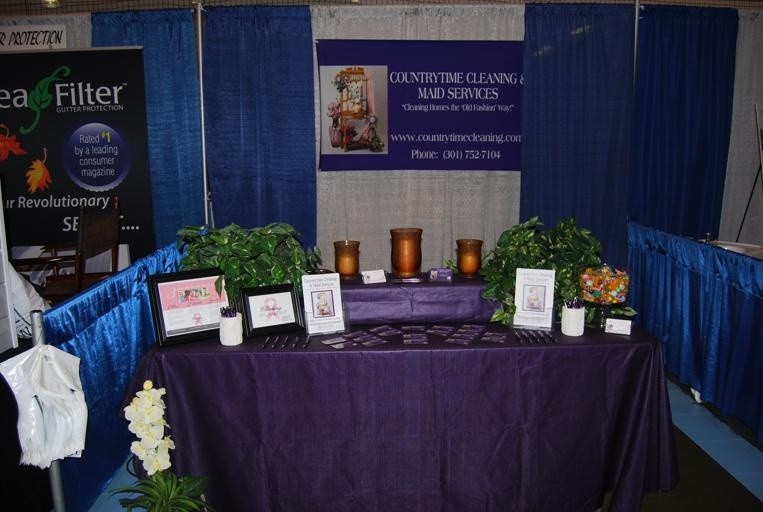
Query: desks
[339,272,506,317]
[148,317,662,512]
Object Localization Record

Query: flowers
[106,382,212,512]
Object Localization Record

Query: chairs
[9,192,119,308]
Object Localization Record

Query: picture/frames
[148,267,232,347]
[242,284,300,338]
